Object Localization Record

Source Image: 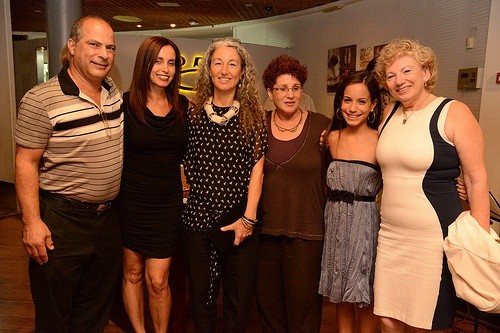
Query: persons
[252,53,338,332]
[317,67,469,332]
[315,34,491,332]
[13,14,126,332]
[116,35,200,333]
[176,37,269,332]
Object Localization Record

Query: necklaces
[269,106,305,133]
[400,92,431,124]
[201,94,240,126]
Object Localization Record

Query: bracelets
[238,214,259,232]
